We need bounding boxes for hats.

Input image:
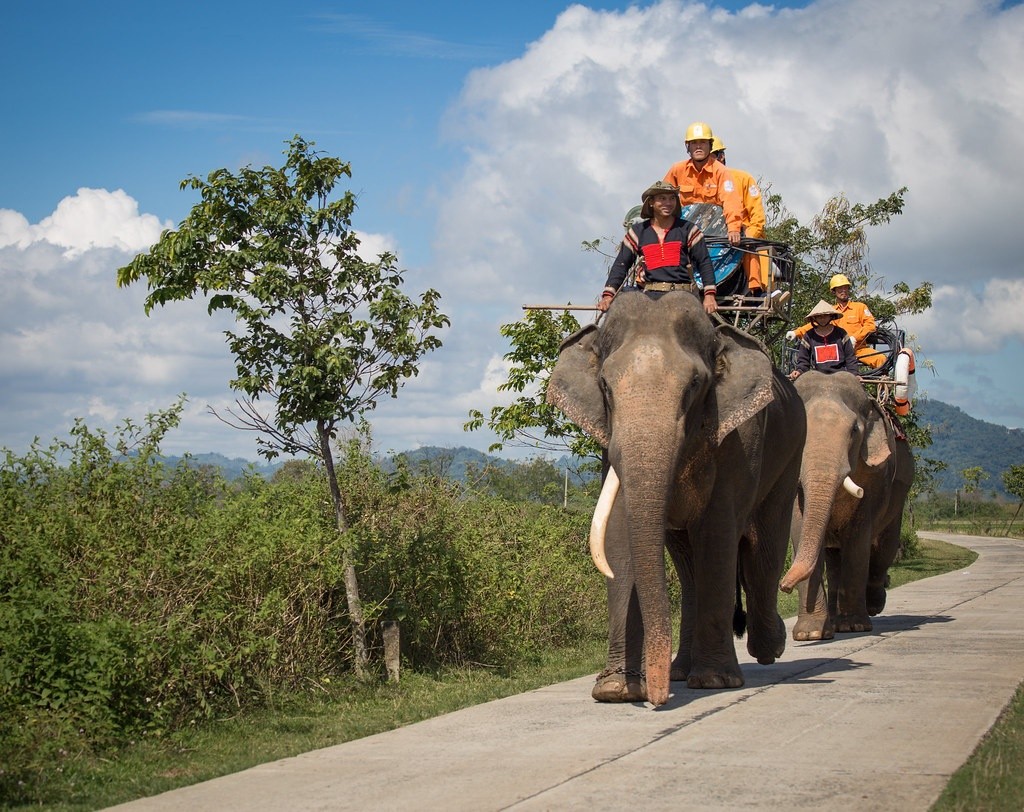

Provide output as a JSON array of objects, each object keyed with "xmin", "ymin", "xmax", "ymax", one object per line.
[
  {"xmin": 640, "ymin": 181, "xmax": 682, "ymax": 218},
  {"xmin": 805, "ymin": 300, "xmax": 843, "ymax": 321}
]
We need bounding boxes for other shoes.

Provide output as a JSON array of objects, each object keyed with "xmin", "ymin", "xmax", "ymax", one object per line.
[
  {"xmin": 881, "ymin": 388, "xmax": 891, "ymax": 400},
  {"xmin": 754, "ymin": 290, "xmax": 781, "ymax": 310},
  {"xmin": 776, "ymin": 291, "xmax": 791, "ymax": 306}
]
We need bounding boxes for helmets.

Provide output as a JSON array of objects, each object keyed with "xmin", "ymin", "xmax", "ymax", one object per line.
[
  {"xmin": 830, "ymin": 275, "xmax": 851, "ymax": 291},
  {"xmin": 684, "ymin": 122, "xmax": 714, "ymax": 142},
  {"xmin": 710, "ymin": 137, "xmax": 726, "ymax": 153}
]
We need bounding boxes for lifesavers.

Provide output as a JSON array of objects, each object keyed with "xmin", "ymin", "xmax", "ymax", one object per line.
[{"xmin": 894, "ymin": 348, "xmax": 916, "ymax": 415}]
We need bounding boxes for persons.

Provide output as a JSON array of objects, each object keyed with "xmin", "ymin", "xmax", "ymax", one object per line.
[
  {"xmin": 709, "ymin": 137, "xmax": 772, "ymax": 293},
  {"xmin": 788, "ymin": 300, "xmax": 863, "ymax": 384},
  {"xmin": 661, "ymin": 122, "xmax": 784, "ymax": 300},
  {"xmin": 785, "ymin": 274, "xmax": 892, "ymax": 397},
  {"xmin": 597, "ymin": 181, "xmax": 719, "ymax": 313}
]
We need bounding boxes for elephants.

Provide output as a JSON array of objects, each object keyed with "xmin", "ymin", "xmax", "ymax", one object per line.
[{"xmin": 544, "ymin": 290, "xmax": 916, "ymax": 706}]
